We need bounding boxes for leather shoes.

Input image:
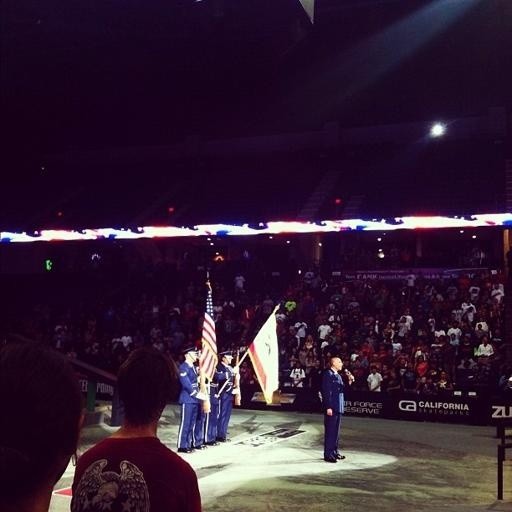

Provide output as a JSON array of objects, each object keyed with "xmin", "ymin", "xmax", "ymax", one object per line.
[
  {"xmin": 335, "ymin": 454, "xmax": 345, "ymax": 459},
  {"xmin": 324, "ymin": 455, "xmax": 337, "ymax": 463},
  {"xmin": 177, "ymin": 437, "xmax": 231, "ymax": 453}
]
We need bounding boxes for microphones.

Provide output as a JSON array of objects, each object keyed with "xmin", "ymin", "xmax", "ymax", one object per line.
[{"xmin": 345, "ymin": 369, "xmax": 355, "ymax": 382}]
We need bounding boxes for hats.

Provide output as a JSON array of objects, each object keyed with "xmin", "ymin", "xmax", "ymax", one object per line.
[
  {"xmin": 182, "ymin": 345, "xmax": 198, "ymax": 355},
  {"xmin": 219, "ymin": 350, "xmax": 233, "ymax": 357}
]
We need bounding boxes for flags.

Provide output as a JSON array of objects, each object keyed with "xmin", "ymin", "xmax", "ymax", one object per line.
[
  {"xmin": 198, "ymin": 282, "xmax": 218, "ymax": 381},
  {"xmin": 248, "ymin": 313, "xmax": 278, "ymax": 403}
]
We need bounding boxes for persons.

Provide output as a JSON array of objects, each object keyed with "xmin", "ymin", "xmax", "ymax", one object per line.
[
  {"xmin": 321, "ymin": 357, "xmax": 344, "ymax": 462},
  {"xmin": 1, "ymin": 330, "xmax": 239, "ymax": 511},
  {"xmin": 2, "ymin": 237, "xmax": 510, "ymax": 390}
]
